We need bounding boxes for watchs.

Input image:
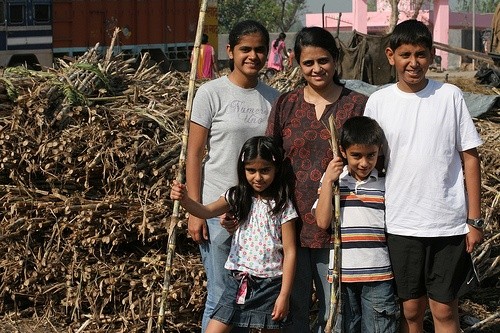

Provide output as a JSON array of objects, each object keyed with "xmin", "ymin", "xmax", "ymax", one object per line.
[{"xmin": 466, "ymin": 217, "xmax": 485, "ymax": 229}]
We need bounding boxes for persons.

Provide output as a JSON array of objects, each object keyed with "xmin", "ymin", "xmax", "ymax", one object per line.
[
  {"xmin": 170, "ymin": 136, "xmax": 300, "ymax": 333},
  {"xmin": 186, "ymin": 20, "xmax": 282, "ymax": 333},
  {"xmin": 221, "ymin": 27, "xmax": 369, "ymax": 333},
  {"xmin": 311, "ymin": 116, "xmax": 398, "ymax": 333},
  {"xmin": 190, "ymin": 32, "xmax": 294, "ymax": 80},
  {"xmin": 362, "ymin": 19, "xmax": 484, "ymax": 333}
]
